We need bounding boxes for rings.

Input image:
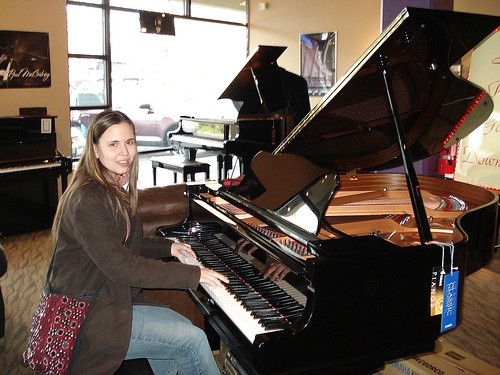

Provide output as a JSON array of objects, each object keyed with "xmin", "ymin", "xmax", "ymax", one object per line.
[
  {"xmin": 243, "ymin": 242, "xmax": 247, "ymax": 245},
  {"xmin": 185, "ymin": 247, "xmax": 189, "ymax": 252},
  {"xmin": 210, "ymin": 271, "xmax": 214, "ymax": 276}
]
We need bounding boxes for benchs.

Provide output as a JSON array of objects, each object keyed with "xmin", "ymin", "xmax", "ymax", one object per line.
[{"xmin": 150, "ymin": 157, "xmax": 211, "ymax": 186}]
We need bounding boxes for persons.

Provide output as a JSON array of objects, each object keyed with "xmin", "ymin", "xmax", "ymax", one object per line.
[
  {"xmin": 41, "ymin": 109, "xmax": 231, "ymax": 375},
  {"xmin": 233, "ymin": 238, "xmax": 291, "ymax": 282}
]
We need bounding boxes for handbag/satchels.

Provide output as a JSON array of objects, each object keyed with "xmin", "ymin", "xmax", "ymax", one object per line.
[{"xmin": 20, "ymin": 281, "xmax": 91, "ymax": 375}]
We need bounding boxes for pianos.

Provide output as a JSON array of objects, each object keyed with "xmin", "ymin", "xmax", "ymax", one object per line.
[
  {"xmin": 163, "ymin": 45, "xmax": 311, "ymax": 180},
  {"xmin": 0, "ymin": 114, "xmax": 73, "ymax": 236},
  {"xmin": 155, "ymin": 6, "xmax": 500, "ymax": 375}
]
